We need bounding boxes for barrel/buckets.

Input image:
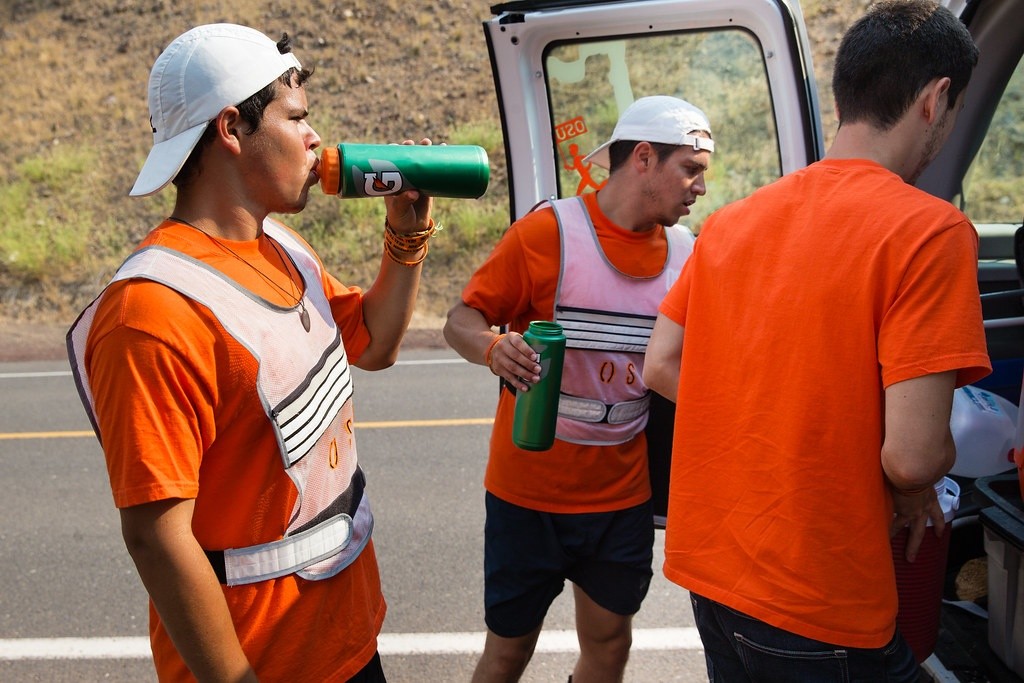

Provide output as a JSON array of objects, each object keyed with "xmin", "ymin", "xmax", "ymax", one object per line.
[{"xmin": 946, "ymin": 384, "xmax": 1019, "ymax": 478}]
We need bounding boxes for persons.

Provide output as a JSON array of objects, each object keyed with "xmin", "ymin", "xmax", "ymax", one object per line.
[
  {"xmin": 442, "ymin": 95, "xmax": 714, "ymax": 683},
  {"xmin": 85, "ymin": 24, "xmax": 447, "ymax": 682},
  {"xmin": 641, "ymin": 0, "xmax": 993, "ymax": 683}
]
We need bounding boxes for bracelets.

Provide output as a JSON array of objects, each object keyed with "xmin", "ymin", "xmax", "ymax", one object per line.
[
  {"xmin": 484, "ymin": 334, "xmax": 506, "ymax": 367},
  {"xmin": 383, "ymin": 216, "xmax": 435, "ymax": 269}
]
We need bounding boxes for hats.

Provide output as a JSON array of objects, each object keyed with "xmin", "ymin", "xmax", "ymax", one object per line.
[
  {"xmin": 582, "ymin": 96, "xmax": 714, "ymax": 170},
  {"xmin": 129, "ymin": 23, "xmax": 303, "ymax": 197}
]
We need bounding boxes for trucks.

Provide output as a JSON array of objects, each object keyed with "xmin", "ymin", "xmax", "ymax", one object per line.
[{"xmin": 483, "ymin": 1, "xmax": 1024, "ymax": 683}]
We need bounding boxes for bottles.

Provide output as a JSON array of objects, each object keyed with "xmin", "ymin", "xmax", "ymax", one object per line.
[
  {"xmin": 314, "ymin": 143, "xmax": 490, "ymax": 198},
  {"xmin": 512, "ymin": 321, "xmax": 566, "ymax": 451}
]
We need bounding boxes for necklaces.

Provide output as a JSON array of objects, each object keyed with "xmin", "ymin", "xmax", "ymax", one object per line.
[{"xmin": 171, "ymin": 215, "xmax": 311, "ymax": 333}]
